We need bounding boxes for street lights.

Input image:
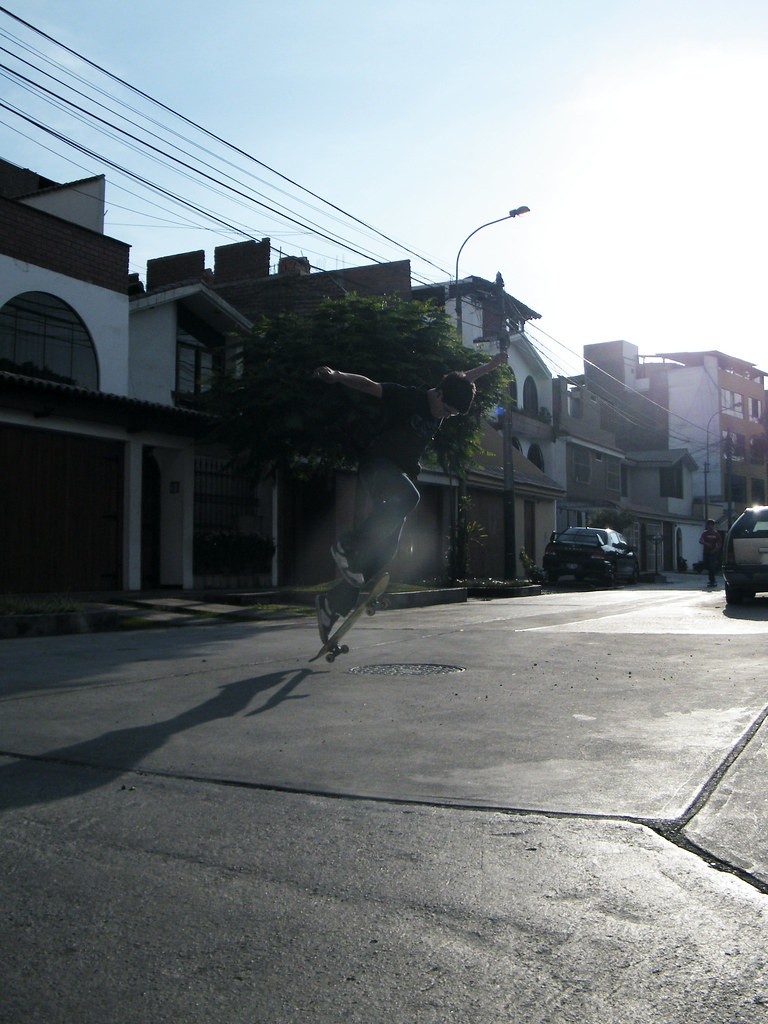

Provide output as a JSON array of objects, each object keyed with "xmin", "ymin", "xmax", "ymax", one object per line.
[
  {"xmin": 703, "ymin": 401, "xmax": 743, "ymax": 531},
  {"xmin": 446, "ymin": 206, "xmax": 529, "ymax": 582}
]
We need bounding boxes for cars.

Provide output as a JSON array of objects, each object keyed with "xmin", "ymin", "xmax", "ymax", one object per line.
[{"xmin": 542, "ymin": 525, "xmax": 641, "ymax": 587}]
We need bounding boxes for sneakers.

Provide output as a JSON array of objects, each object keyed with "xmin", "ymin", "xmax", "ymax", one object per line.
[
  {"xmin": 331, "ymin": 543, "xmax": 366, "ymax": 588},
  {"xmin": 315, "ymin": 593, "xmax": 340, "ymax": 644}
]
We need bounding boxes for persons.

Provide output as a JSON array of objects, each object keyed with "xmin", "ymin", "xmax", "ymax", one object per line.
[
  {"xmin": 699, "ymin": 519, "xmax": 723, "ymax": 584},
  {"xmin": 312, "ymin": 352, "xmax": 509, "ymax": 646}
]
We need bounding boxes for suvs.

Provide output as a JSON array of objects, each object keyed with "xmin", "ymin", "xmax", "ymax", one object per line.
[{"xmin": 721, "ymin": 506, "xmax": 768, "ymax": 605}]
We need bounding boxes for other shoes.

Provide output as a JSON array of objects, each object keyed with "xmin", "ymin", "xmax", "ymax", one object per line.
[{"xmin": 708, "ymin": 582, "xmax": 717, "ymax": 587}]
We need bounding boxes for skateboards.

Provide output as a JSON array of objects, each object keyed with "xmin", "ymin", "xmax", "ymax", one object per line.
[
  {"xmin": 707, "ymin": 581, "xmax": 717, "ymax": 587},
  {"xmin": 307, "ymin": 572, "xmax": 390, "ymax": 663}
]
